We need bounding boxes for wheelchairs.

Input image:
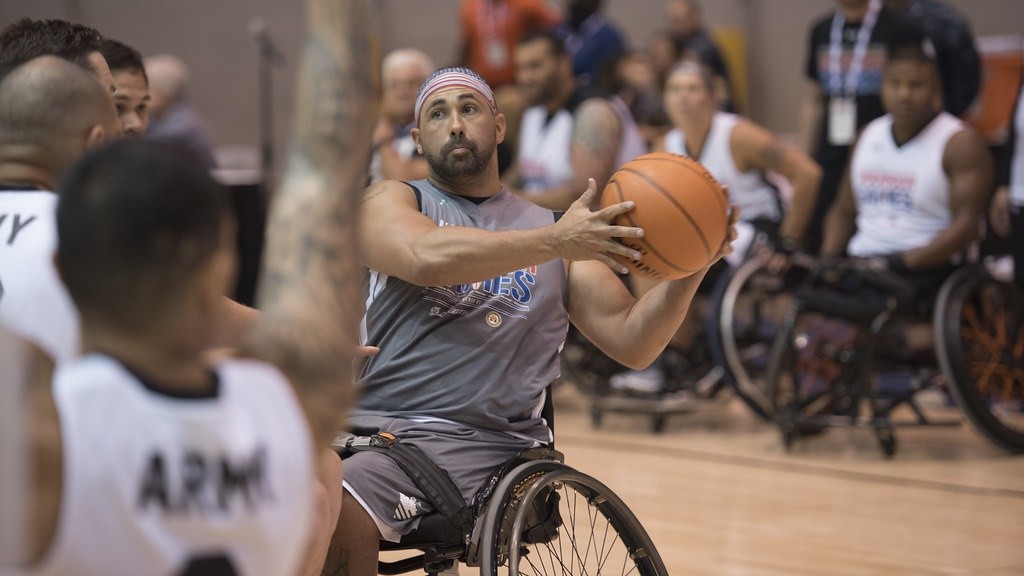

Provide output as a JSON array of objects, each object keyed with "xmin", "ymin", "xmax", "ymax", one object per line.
[
  {"xmin": 369, "ymin": 383, "xmax": 669, "ymax": 576},
  {"xmin": 589, "ymin": 217, "xmax": 1024, "ymax": 457}
]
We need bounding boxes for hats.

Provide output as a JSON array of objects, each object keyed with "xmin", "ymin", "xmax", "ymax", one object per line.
[{"xmin": 415, "ymin": 68, "xmax": 498, "ymax": 130}]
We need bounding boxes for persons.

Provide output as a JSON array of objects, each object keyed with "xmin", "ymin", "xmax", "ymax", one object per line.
[{"xmin": 0, "ymin": 0, "xmax": 1024, "ymax": 576}]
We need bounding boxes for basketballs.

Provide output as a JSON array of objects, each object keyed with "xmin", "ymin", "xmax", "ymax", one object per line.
[{"xmin": 598, "ymin": 150, "xmax": 729, "ymax": 283}]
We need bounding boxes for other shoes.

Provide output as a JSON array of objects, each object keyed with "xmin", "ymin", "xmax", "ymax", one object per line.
[{"xmin": 609, "ymin": 368, "xmax": 666, "ymax": 396}]
